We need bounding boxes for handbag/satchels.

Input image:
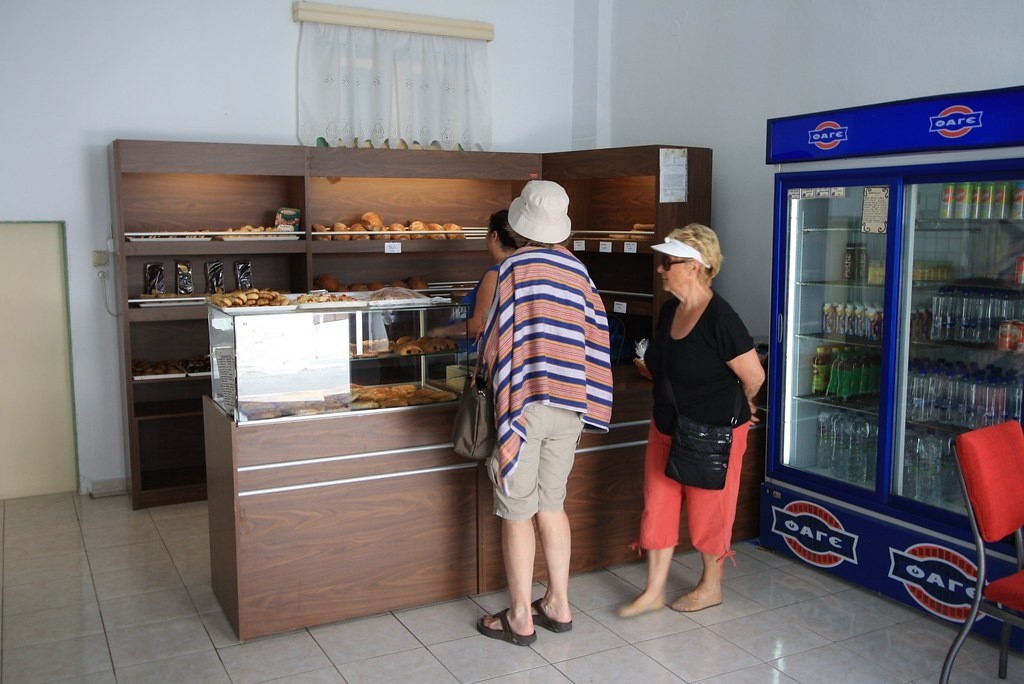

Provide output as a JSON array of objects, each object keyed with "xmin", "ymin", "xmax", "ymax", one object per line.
[
  {"xmin": 453, "ymin": 376, "xmax": 496, "ymax": 461},
  {"xmin": 664, "ymin": 414, "xmax": 734, "ymax": 491}
]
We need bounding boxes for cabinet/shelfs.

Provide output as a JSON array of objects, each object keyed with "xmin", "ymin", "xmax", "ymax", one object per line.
[{"xmin": 108, "ymin": 139, "xmax": 711, "ymax": 513}]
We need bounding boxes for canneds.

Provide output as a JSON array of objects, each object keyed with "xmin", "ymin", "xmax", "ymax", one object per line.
[
  {"xmin": 1013, "ymin": 256, "xmax": 1024, "ymax": 287},
  {"xmin": 939, "ymin": 181, "xmax": 1024, "ymax": 220},
  {"xmin": 997, "ymin": 319, "xmax": 1024, "ymax": 352}
]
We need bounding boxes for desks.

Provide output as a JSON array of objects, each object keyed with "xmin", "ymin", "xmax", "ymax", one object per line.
[
  {"xmin": 426, "ymin": 374, "xmax": 762, "ymax": 595},
  {"xmin": 201, "ymin": 381, "xmax": 479, "ymax": 642}
]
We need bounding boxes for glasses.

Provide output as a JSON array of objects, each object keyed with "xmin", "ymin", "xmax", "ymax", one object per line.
[{"xmin": 660, "ymin": 253, "xmax": 686, "ymax": 271}]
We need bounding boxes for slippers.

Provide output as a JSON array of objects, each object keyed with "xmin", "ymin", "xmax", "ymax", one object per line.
[
  {"xmin": 531, "ymin": 598, "xmax": 573, "ymax": 633},
  {"xmin": 476, "ymin": 608, "xmax": 537, "ymax": 646}
]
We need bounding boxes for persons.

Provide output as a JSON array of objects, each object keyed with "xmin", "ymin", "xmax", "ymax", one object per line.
[
  {"xmin": 615, "ymin": 225, "xmax": 765, "ymax": 617},
  {"xmin": 424, "ymin": 209, "xmax": 520, "ymax": 381},
  {"xmin": 476, "ymin": 179, "xmax": 612, "ymax": 646}
]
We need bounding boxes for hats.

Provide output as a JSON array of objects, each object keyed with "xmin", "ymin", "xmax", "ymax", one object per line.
[
  {"xmin": 508, "ymin": 180, "xmax": 572, "ymax": 243},
  {"xmin": 650, "ymin": 237, "xmax": 711, "ymax": 269}
]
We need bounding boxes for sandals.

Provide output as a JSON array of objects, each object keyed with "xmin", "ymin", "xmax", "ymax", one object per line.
[
  {"xmin": 617, "ymin": 595, "xmax": 665, "ymax": 618},
  {"xmin": 671, "ymin": 590, "xmax": 723, "ymax": 612}
]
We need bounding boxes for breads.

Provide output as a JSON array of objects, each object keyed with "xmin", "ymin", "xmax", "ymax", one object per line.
[
  {"xmin": 132, "ymin": 212, "xmax": 477, "ymax": 420},
  {"xmin": 609, "ymin": 223, "xmax": 655, "ymax": 240}
]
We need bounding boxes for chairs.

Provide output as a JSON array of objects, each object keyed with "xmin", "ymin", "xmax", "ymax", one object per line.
[{"xmin": 938, "ymin": 420, "xmax": 1024, "ymax": 683}]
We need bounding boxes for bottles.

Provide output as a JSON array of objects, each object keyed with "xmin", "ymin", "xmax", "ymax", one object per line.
[
  {"xmin": 844, "ymin": 243, "xmax": 868, "ymax": 284},
  {"xmin": 823, "ymin": 302, "xmax": 931, "ymax": 343},
  {"xmin": 906, "ymin": 357, "xmax": 1024, "ymax": 429},
  {"xmin": 812, "ymin": 347, "xmax": 881, "ymax": 403},
  {"xmin": 930, "ymin": 286, "xmax": 1024, "ymax": 345},
  {"xmin": 904, "ymin": 423, "xmax": 964, "ymax": 506},
  {"xmin": 816, "ymin": 406, "xmax": 879, "ymax": 483}
]
